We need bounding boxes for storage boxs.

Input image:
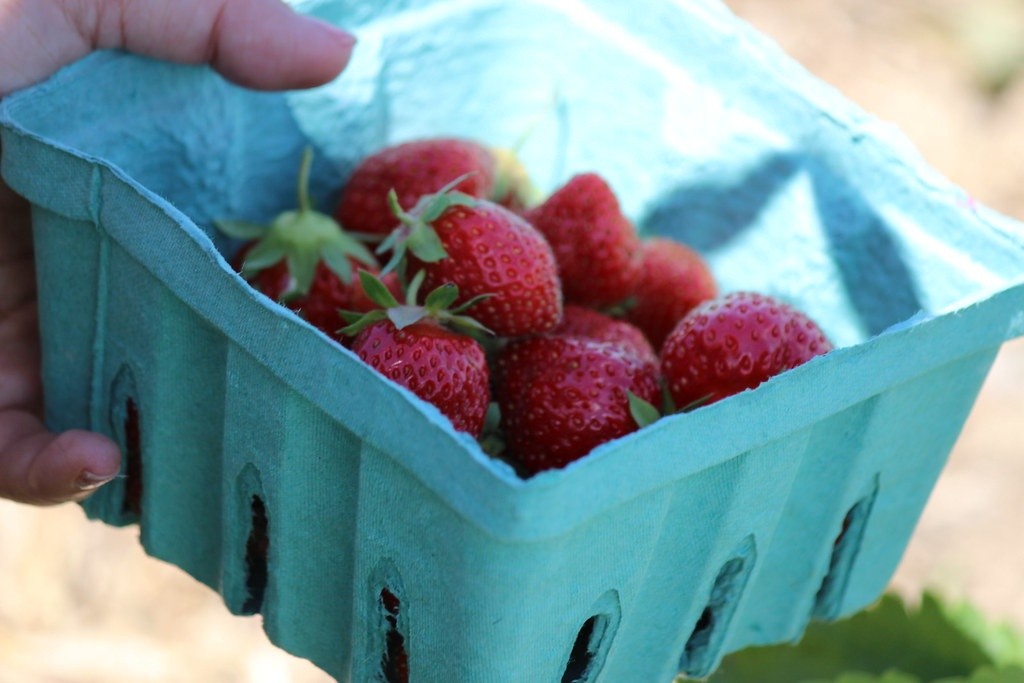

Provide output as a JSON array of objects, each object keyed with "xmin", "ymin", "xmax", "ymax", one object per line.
[{"xmin": 0, "ymin": 0, "xmax": 1024, "ymax": 683}]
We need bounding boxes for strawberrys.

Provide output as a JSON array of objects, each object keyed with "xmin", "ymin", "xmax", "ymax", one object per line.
[{"xmin": 210, "ymin": 139, "xmax": 840, "ymax": 481}]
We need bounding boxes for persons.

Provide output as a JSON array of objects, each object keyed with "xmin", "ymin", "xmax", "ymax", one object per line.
[{"xmin": 0, "ymin": 0, "xmax": 360, "ymax": 509}]
